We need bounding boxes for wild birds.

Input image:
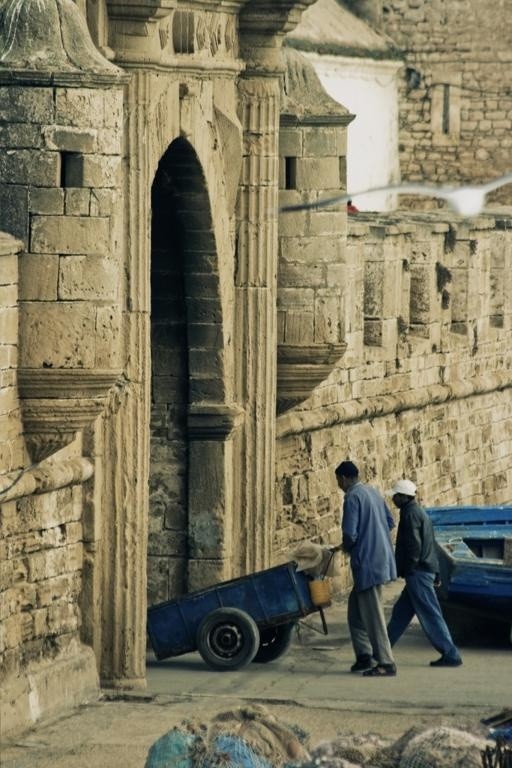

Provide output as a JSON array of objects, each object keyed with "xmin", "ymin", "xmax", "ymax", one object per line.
[{"xmin": 277, "ymin": 173, "xmax": 512, "ymax": 222}]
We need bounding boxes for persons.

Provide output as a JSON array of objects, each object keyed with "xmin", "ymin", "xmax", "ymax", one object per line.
[
  {"xmin": 334, "ymin": 457, "xmax": 402, "ymax": 678},
  {"xmin": 369, "ymin": 477, "xmax": 463, "ymax": 667}
]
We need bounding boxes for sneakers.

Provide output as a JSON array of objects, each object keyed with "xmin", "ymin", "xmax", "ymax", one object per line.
[
  {"xmin": 350, "ymin": 657, "xmax": 373, "ymax": 672},
  {"xmin": 363, "ymin": 666, "xmax": 397, "ymax": 677},
  {"xmin": 430, "ymin": 655, "xmax": 462, "ymax": 667}
]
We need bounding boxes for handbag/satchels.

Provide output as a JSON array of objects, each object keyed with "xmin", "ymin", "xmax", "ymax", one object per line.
[{"xmin": 308, "ymin": 580, "xmax": 334, "ymax": 606}]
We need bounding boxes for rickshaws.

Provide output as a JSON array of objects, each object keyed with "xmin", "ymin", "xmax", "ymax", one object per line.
[{"xmin": 147, "ymin": 544, "xmax": 343, "ymax": 671}]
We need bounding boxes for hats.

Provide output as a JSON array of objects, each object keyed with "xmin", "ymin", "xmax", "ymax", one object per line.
[{"xmin": 384, "ymin": 479, "xmax": 417, "ymax": 498}]
